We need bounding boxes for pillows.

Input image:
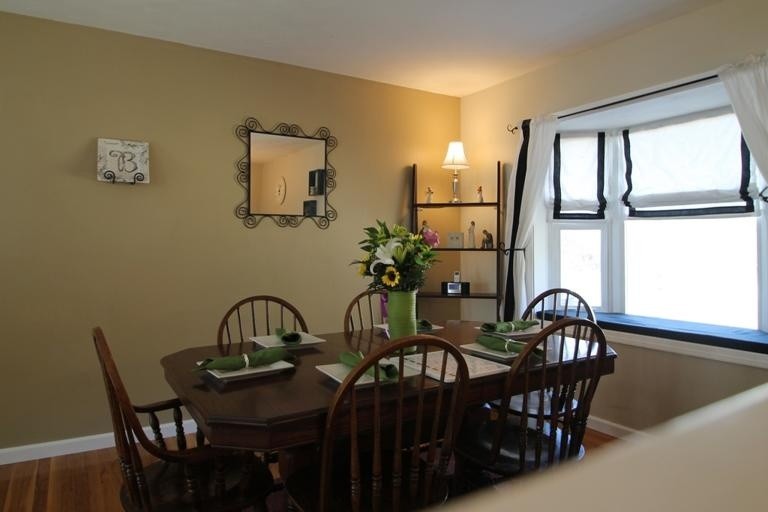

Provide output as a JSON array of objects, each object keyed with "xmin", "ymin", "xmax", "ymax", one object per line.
[
  {"xmin": 192, "ymin": 347, "xmax": 288, "ymax": 372},
  {"xmin": 417, "ymin": 318, "xmax": 432, "ymax": 331},
  {"xmin": 477, "ymin": 336, "xmax": 547, "ymax": 362},
  {"xmin": 277, "ymin": 328, "xmax": 302, "ymax": 344},
  {"xmin": 481, "ymin": 321, "xmax": 539, "ymax": 333},
  {"xmin": 340, "ymin": 349, "xmax": 398, "ymax": 379}
]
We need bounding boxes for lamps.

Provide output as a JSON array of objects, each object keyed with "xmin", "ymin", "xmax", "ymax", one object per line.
[{"xmin": 442, "ymin": 142, "xmax": 469, "ymax": 202}]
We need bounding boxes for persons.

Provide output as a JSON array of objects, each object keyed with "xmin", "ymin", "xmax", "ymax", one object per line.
[
  {"xmin": 468, "ymin": 221, "xmax": 476, "ymax": 249},
  {"xmin": 420, "ymin": 220, "xmax": 439, "ymax": 248},
  {"xmin": 479, "ymin": 230, "xmax": 493, "ymax": 249},
  {"xmin": 425, "ymin": 186, "xmax": 434, "ymax": 202},
  {"xmin": 476, "ymin": 186, "xmax": 483, "ymax": 203}
]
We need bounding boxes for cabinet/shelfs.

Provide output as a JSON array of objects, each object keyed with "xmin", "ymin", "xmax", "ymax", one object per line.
[{"xmin": 410, "ymin": 161, "xmax": 504, "ymax": 321}]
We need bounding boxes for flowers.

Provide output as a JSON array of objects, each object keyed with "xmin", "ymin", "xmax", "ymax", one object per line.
[{"xmin": 350, "ymin": 218, "xmax": 437, "ymax": 291}]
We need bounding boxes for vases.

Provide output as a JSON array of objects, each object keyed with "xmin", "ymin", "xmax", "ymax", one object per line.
[{"xmin": 387, "ymin": 292, "xmax": 418, "ymax": 352}]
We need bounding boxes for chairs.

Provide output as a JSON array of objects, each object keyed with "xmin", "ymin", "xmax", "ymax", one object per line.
[
  {"xmin": 489, "ymin": 289, "xmax": 592, "ymax": 418},
  {"xmin": 458, "ymin": 317, "xmax": 606, "ymax": 494},
  {"xmin": 283, "ymin": 334, "xmax": 469, "ymax": 512},
  {"xmin": 92, "ymin": 327, "xmax": 274, "ymax": 512},
  {"xmin": 345, "ymin": 291, "xmax": 380, "ymax": 331},
  {"xmin": 217, "ymin": 296, "xmax": 308, "ymax": 491}
]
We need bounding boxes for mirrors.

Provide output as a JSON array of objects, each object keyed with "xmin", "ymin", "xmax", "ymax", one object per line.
[{"xmin": 234, "ymin": 117, "xmax": 337, "ymax": 228}]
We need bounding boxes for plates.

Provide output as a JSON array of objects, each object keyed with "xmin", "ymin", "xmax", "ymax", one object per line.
[
  {"xmin": 315, "ymin": 357, "xmax": 423, "ymax": 389},
  {"xmin": 472, "ymin": 325, "xmax": 545, "ymax": 339},
  {"xmin": 248, "ymin": 331, "xmax": 328, "ymax": 352},
  {"xmin": 195, "ymin": 353, "xmax": 298, "ymax": 384},
  {"xmin": 373, "ymin": 321, "xmax": 446, "ymax": 335},
  {"xmin": 459, "ymin": 341, "xmax": 553, "ymax": 363}
]
